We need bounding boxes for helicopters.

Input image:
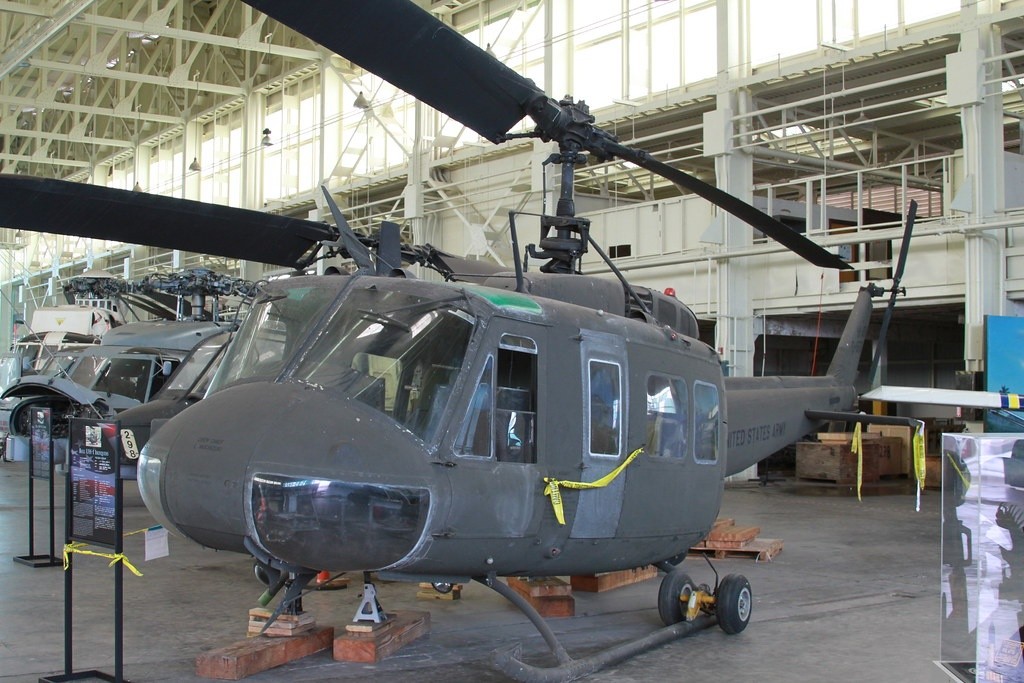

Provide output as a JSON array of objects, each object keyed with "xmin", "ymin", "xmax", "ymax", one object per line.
[{"xmin": 0, "ymin": 0, "xmax": 1024, "ymax": 683}]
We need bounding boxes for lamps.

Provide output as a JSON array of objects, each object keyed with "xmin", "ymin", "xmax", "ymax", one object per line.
[
  {"xmin": 354, "ymin": 68, "xmax": 368, "ymax": 108},
  {"xmin": 189, "ymin": 75, "xmax": 201, "ymax": 171},
  {"xmin": 132, "ymin": 112, "xmax": 142, "ymax": 191},
  {"xmin": 261, "ymin": 36, "xmax": 273, "ymax": 145}
]
